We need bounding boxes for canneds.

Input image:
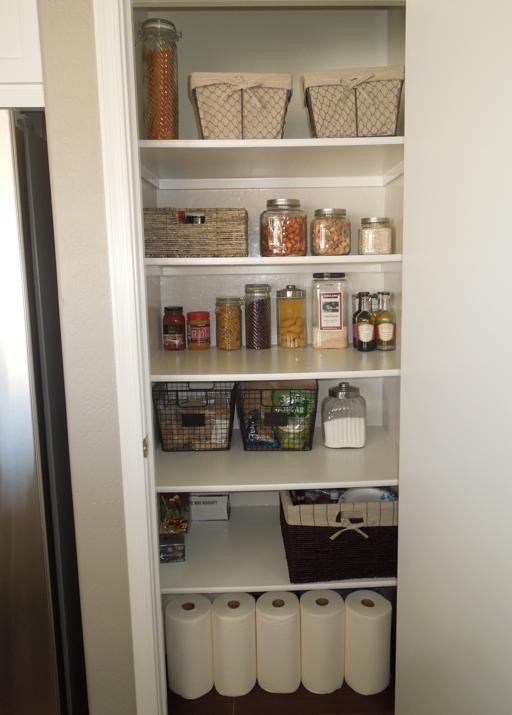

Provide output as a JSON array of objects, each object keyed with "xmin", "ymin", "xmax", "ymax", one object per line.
[{"xmin": 187, "ymin": 312, "xmax": 211, "ymax": 347}]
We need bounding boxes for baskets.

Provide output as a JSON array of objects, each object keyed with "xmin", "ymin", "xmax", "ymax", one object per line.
[
  {"xmin": 152, "ymin": 382, "xmax": 235, "ymax": 452},
  {"xmin": 188, "ymin": 72, "xmax": 293, "ymax": 138},
  {"xmin": 236, "ymin": 380, "xmax": 318, "ymax": 451},
  {"xmin": 301, "ymin": 65, "xmax": 404, "ymax": 138}
]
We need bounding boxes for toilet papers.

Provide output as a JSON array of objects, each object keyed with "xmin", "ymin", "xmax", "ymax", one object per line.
[
  {"xmin": 211, "ymin": 593, "xmax": 257, "ymax": 698},
  {"xmin": 163, "ymin": 594, "xmax": 214, "ymax": 701},
  {"xmin": 345, "ymin": 589, "xmax": 393, "ymax": 696},
  {"xmin": 255, "ymin": 591, "xmax": 302, "ymax": 696},
  {"xmin": 299, "ymin": 590, "xmax": 345, "ymax": 695}
]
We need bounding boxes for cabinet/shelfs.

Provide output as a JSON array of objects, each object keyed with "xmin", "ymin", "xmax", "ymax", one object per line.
[{"xmin": 91, "ymin": 2, "xmax": 406, "ymax": 715}]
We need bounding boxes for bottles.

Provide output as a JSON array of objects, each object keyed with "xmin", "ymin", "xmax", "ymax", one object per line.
[
  {"xmin": 215, "ymin": 296, "xmax": 242, "ymax": 351},
  {"xmin": 244, "ymin": 284, "xmax": 272, "ymax": 351},
  {"xmin": 321, "ymin": 382, "xmax": 366, "ymax": 451},
  {"xmin": 352, "ymin": 288, "xmax": 397, "ymax": 353},
  {"xmin": 276, "ymin": 284, "xmax": 308, "ymax": 351},
  {"xmin": 134, "ymin": 20, "xmax": 182, "ymax": 140},
  {"xmin": 259, "ymin": 198, "xmax": 308, "ymax": 257},
  {"xmin": 310, "ymin": 206, "xmax": 352, "ymax": 255},
  {"xmin": 357, "ymin": 215, "xmax": 394, "ymax": 255},
  {"xmin": 185, "ymin": 310, "xmax": 212, "ymax": 350},
  {"xmin": 311, "ymin": 271, "xmax": 351, "ymax": 351}
]
